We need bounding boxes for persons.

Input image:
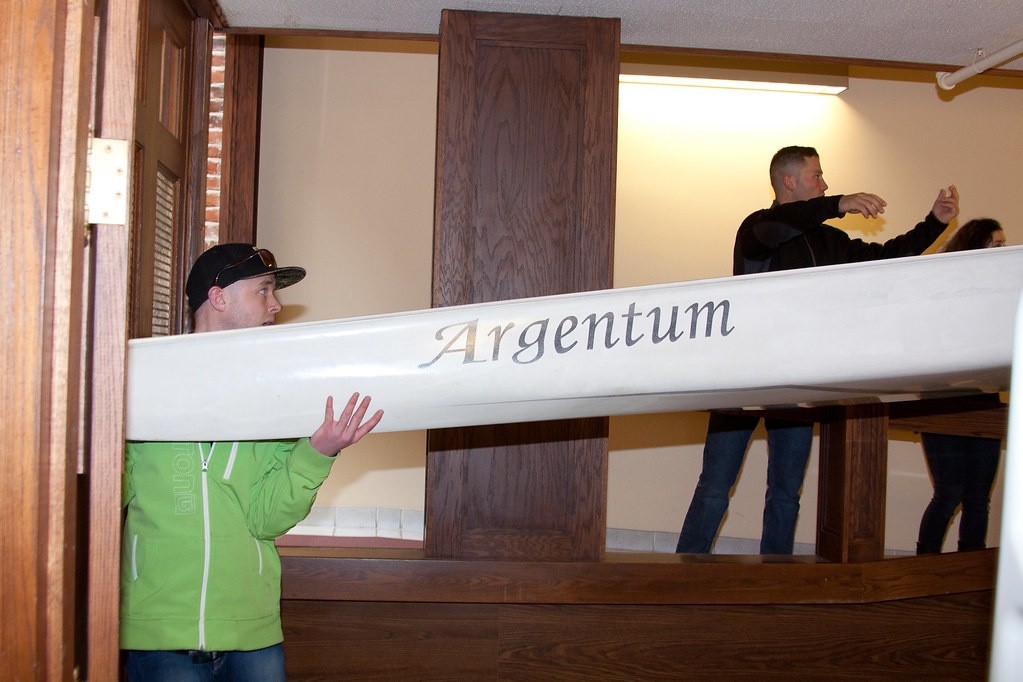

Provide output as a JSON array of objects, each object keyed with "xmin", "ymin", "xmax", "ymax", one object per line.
[
  {"xmin": 116, "ymin": 242, "xmax": 383, "ymax": 682},
  {"xmin": 676, "ymin": 145, "xmax": 959, "ymax": 555},
  {"xmin": 918, "ymin": 219, "xmax": 1006, "ymax": 556}
]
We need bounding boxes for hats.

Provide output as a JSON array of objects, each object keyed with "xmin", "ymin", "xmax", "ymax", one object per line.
[{"xmin": 186, "ymin": 243, "xmax": 306, "ymax": 313}]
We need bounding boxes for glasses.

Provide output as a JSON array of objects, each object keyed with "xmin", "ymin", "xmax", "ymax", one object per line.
[{"xmin": 215, "ymin": 249, "xmax": 277, "ymax": 285}]
